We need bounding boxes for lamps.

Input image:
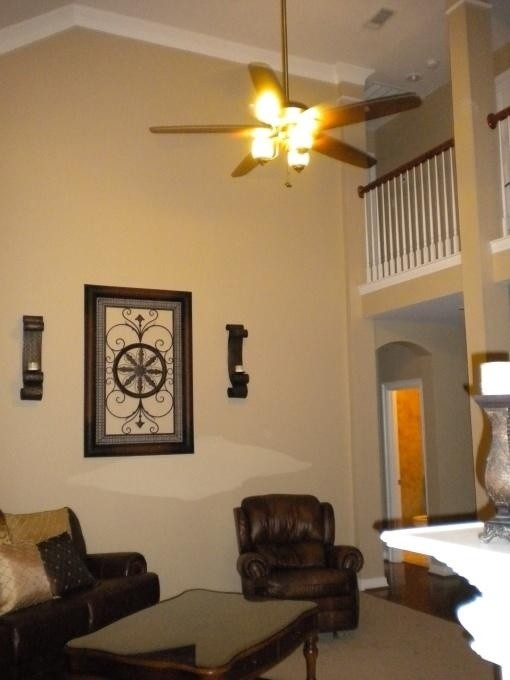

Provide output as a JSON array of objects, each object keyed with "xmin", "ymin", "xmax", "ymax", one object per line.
[
  {"xmin": 251, "ymin": 0, "xmax": 322, "ymax": 174},
  {"xmin": 20, "ymin": 315, "xmax": 249, "ymax": 400}
]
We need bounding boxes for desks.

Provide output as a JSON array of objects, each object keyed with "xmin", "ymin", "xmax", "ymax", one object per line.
[{"xmin": 380, "ymin": 521, "xmax": 510, "ymax": 680}]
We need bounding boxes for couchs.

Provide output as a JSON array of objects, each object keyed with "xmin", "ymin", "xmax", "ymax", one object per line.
[{"xmin": 0, "ymin": 507, "xmax": 160, "ymax": 680}]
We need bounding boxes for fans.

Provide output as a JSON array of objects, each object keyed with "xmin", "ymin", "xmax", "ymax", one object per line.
[{"xmin": 151, "ymin": 0, "xmax": 422, "ymax": 178}]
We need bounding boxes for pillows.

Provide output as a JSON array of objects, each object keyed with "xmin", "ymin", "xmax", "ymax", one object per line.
[{"xmin": 0, "ymin": 533, "xmax": 99, "ymax": 616}]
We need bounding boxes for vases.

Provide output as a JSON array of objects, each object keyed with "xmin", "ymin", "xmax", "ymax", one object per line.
[{"xmin": 475, "ymin": 395, "xmax": 510, "ymax": 545}]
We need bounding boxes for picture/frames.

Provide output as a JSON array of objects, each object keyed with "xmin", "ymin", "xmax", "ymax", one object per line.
[{"xmin": 84, "ymin": 282, "xmax": 193, "ymax": 457}]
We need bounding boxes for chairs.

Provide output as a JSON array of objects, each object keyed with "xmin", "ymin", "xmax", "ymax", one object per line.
[{"xmin": 233, "ymin": 495, "xmax": 365, "ymax": 635}]
